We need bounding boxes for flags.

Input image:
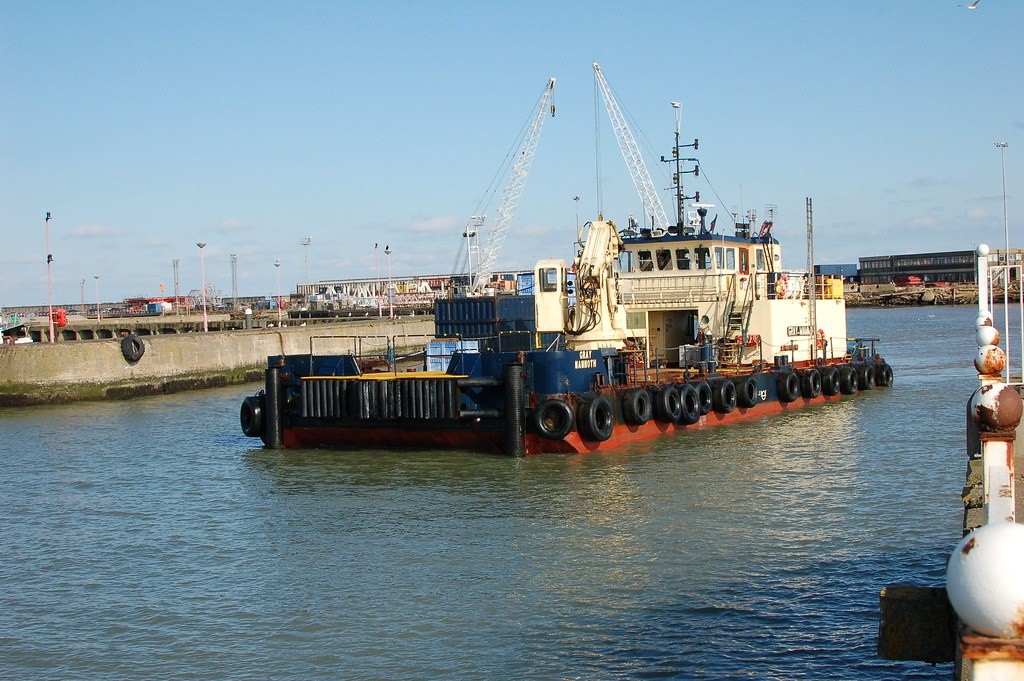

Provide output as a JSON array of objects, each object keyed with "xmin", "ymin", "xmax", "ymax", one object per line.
[{"xmin": 709, "ymin": 217, "xmax": 716, "ymax": 232}]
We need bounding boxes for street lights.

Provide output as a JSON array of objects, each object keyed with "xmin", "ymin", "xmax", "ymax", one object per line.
[
  {"xmin": 94, "ymin": 276, "xmax": 100, "ymax": 322},
  {"xmin": 45, "ymin": 212, "xmax": 54, "ymax": 343},
  {"xmin": 274, "ymin": 263, "xmax": 281, "ymax": 328},
  {"xmin": 574, "ymin": 197, "xmax": 580, "ymax": 230},
  {"xmin": 384, "ymin": 245, "xmax": 393, "ymax": 318},
  {"xmin": 375, "ymin": 243, "xmax": 382, "ymax": 317},
  {"xmin": 197, "ymin": 242, "xmax": 207, "ymax": 332},
  {"xmin": 996, "ymin": 142, "xmax": 1010, "ymax": 284}
]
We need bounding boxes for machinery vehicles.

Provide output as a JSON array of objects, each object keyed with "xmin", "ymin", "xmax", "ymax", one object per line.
[
  {"xmin": 451, "ymin": 77, "xmax": 556, "ymax": 298},
  {"xmin": 592, "ymin": 62, "xmax": 699, "ymax": 272}
]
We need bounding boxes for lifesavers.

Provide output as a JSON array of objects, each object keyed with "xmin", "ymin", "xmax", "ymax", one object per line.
[
  {"xmin": 577, "ymin": 395, "xmax": 614, "ymax": 443},
  {"xmin": 655, "ymin": 375, "xmax": 761, "ymax": 426},
  {"xmin": 120, "ymin": 334, "xmax": 147, "ymax": 362},
  {"xmin": 531, "ymin": 399, "xmax": 574, "ymax": 440},
  {"xmin": 816, "ymin": 328, "xmax": 825, "ymax": 349},
  {"xmin": 239, "ymin": 395, "xmax": 261, "ymax": 438},
  {"xmin": 775, "ymin": 362, "xmax": 894, "ymax": 404},
  {"xmin": 621, "ymin": 388, "xmax": 652, "ymax": 427}
]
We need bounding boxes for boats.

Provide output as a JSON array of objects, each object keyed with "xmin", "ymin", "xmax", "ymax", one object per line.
[{"xmin": 240, "ymin": 101, "xmax": 893, "ymax": 458}]
